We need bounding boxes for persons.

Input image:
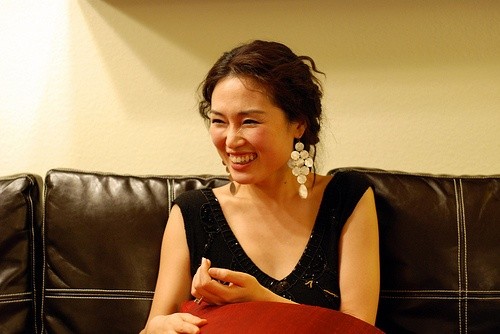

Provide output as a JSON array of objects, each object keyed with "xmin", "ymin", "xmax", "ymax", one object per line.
[{"xmin": 137, "ymin": 40, "xmax": 380, "ymax": 334}]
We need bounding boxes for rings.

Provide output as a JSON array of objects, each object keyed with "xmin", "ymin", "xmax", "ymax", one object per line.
[{"xmin": 194, "ymin": 296, "xmax": 205, "ymax": 305}]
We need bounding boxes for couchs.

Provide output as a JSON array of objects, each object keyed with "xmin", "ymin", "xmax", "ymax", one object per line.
[{"xmin": 0, "ymin": 167, "xmax": 500, "ymax": 334}]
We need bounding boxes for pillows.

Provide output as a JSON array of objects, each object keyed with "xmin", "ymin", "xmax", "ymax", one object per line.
[{"xmin": 177, "ymin": 299, "xmax": 386, "ymax": 334}]
